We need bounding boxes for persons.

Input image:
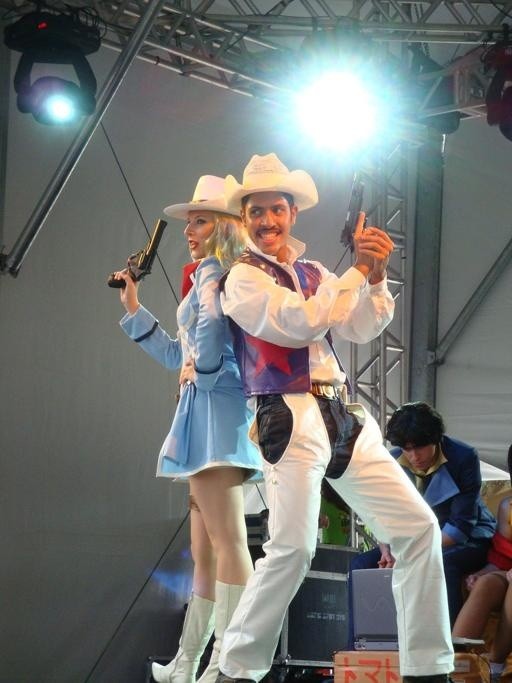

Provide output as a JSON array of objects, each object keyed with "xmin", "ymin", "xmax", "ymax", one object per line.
[
  {"xmin": 449, "ymin": 439, "xmax": 511, "ymax": 682},
  {"xmin": 362, "ymin": 400, "xmax": 496, "ymax": 627},
  {"xmin": 213, "ymin": 151, "xmax": 456, "ymax": 683},
  {"xmin": 111, "ymin": 174, "xmax": 263, "ymax": 683}
]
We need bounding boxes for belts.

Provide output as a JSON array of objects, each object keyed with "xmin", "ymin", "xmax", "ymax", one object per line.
[{"xmin": 310, "ymin": 382, "xmax": 346, "ymax": 405}]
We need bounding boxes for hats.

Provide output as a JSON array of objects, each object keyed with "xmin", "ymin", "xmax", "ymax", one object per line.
[
  {"xmin": 163, "ymin": 175, "xmax": 242, "ymax": 220},
  {"xmin": 223, "ymin": 152, "xmax": 319, "ymax": 216}
]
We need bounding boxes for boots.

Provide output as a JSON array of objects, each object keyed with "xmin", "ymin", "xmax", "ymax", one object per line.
[
  {"xmin": 196, "ymin": 579, "xmax": 246, "ymax": 683},
  {"xmin": 151, "ymin": 591, "xmax": 216, "ymax": 683}
]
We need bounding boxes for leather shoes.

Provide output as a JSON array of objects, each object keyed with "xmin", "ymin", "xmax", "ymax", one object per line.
[
  {"xmin": 215, "ymin": 671, "xmax": 256, "ymax": 683},
  {"xmin": 402, "ymin": 674, "xmax": 454, "ymax": 683}
]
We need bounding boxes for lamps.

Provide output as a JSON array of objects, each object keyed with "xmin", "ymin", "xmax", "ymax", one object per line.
[{"xmin": 4, "ymin": 10, "xmax": 100, "ymax": 128}]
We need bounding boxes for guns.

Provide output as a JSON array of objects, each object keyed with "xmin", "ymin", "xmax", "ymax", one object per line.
[
  {"xmin": 338, "ymin": 175, "xmax": 366, "ymax": 248},
  {"xmin": 108, "ymin": 220, "xmax": 166, "ymax": 289}
]
